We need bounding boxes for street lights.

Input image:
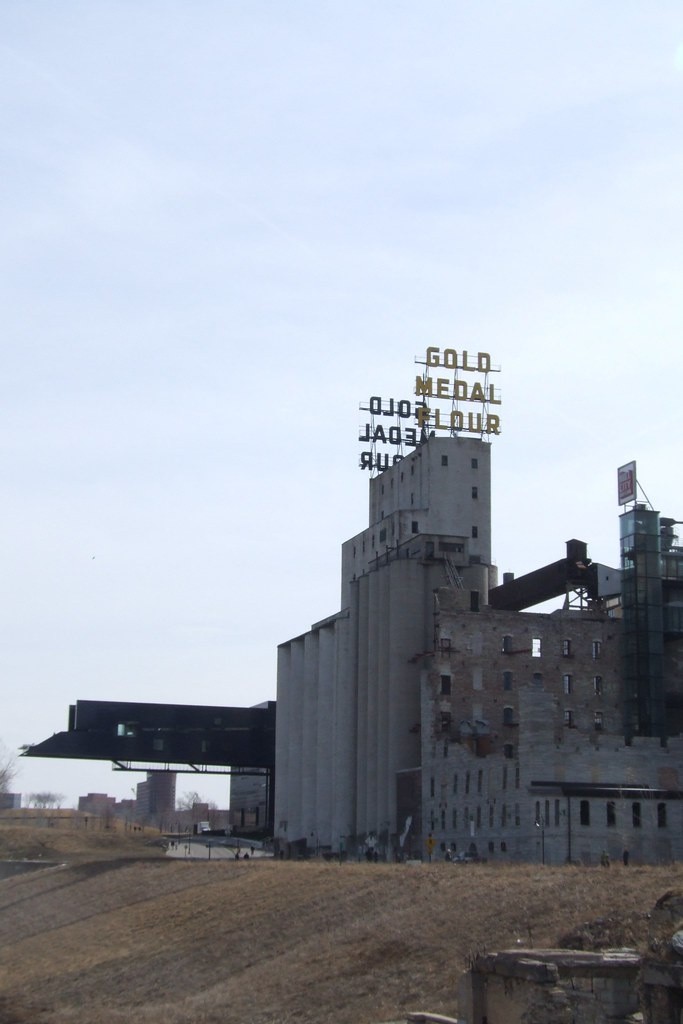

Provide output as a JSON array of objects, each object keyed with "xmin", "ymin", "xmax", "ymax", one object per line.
[
  {"xmin": 311, "ymin": 828, "xmax": 319, "ymax": 856},
  {"xmin": 535, "ymin": 817, "xmax": 546, "ymax": 866}
]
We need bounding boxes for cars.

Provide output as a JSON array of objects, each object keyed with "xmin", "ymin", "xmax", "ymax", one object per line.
[{"xmin": 452, "ymin": 851, "xmax": 486, "ymax": 864}]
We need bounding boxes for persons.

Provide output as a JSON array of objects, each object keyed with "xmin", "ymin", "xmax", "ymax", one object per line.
[
  {"xmin": 234, "ymin": 845, "xmax": 256, "ymax": 860},
  {"xmin": 623, "ymin": 846, "xmax": 629, "ymax": 866}
]
